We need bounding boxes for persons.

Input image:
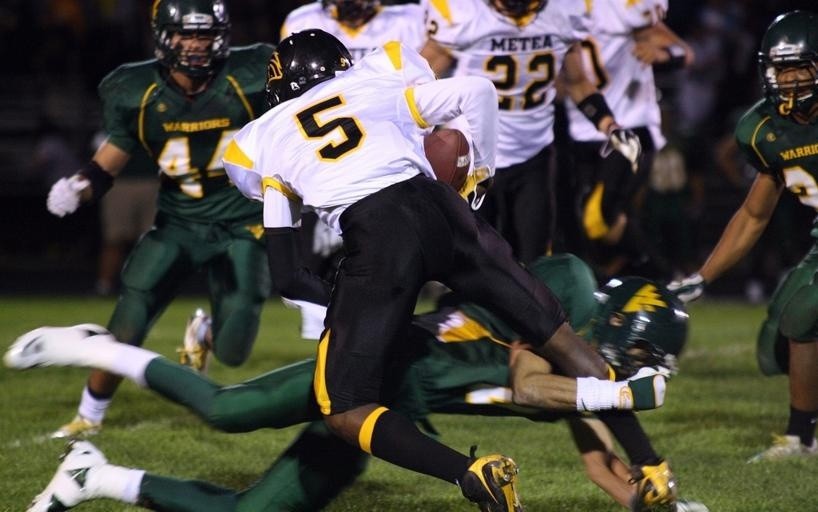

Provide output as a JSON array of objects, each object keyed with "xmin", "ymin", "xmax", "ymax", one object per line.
[
  {"xmin": 0, "ymin": 252, "xmax": 692, "ymax": 508},
  {"xmin": 219, "ymin": 25, "xmax": 682, "ymax": 508},
  {"xmin": 1, "ymin": 0, "xmax": 816, "ymax": 470}
]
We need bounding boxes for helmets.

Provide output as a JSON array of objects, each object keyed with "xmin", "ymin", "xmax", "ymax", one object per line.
[
  {"xmin": 151, "ymin": 0, "xmax": 231, "ymax": 78},
  {"xmin": 266, "ymin": 29, "xmax": 353, "ymax": 109},
  {"xmin": 595, "ymin": 278, "xmax": 687, "ymax": 371},
  {"xmin": 759, "ymin": 10, "xmax": 818, "ymax": 117}
]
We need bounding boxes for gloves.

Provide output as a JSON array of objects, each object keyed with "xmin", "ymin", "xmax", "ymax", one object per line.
[
  {"xmin": 600, "ymin": 127, "xmax": 641, "ymax": 162},
  {"xmin": 628, "ymin": 366, "xmax": 665, "ymax": 410}
]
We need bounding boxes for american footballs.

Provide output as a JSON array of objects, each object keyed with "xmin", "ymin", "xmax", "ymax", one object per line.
[{"xmin": 423, "ymin": 127, "xmax": 471, "ymax": 192}]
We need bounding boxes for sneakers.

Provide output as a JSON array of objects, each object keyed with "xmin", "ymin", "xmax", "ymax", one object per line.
[
  {"xmin": 179, "ymin": 309, "xmax": 211, "ymax": 371},
  {"xmin": 4, "ymin": 323, "xmax": 110, "ymax": 371},
  {"xmin": 27, "ymin": 414, "xmax": 106, "ymax": 512},
  {"xmin": 628, "ymin": 460, "xmax": 677, "ymax": 512},
  {"xmin": 47, "ymin": 176, "xmax": 81, "ymax": 217},
  {"xmin": 747, "ymin": 435, "xmax": 817, "ymax": 464},
  {"xmin": 458, "ymin": 445, "xmax": 523, "ymax": 512}
]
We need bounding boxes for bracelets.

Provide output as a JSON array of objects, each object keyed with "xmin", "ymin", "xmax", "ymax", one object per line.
[{"xmin": 572, "ymin": 374, "xmax": 622, "ymax": 421}]
